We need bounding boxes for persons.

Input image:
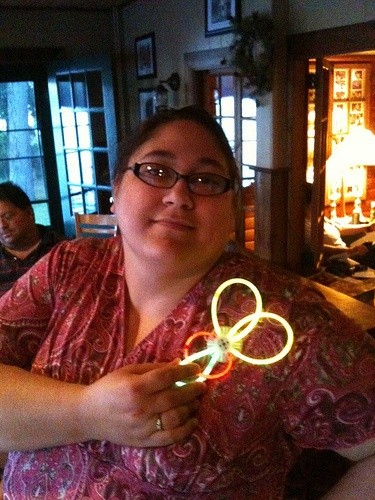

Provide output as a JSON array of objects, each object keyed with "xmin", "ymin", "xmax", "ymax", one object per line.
[
  {"xmin": 0, "ymin": 107, "xmax": 374, "ymax": 500},
  {"xmin": 0, "ymin": 181, "xmax": 67, "ymax": 299}
]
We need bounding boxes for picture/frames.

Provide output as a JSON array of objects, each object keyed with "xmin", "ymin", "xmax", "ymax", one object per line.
[
  {"xmin": 204, "ymin": 0, "xmax": 240, "ymax": 35},
  {"xmin": 138, "ymin": 88, "xmax": 159, "ymax": 124},
  {"xmin": 134, "ymin": 31, "xmax": 156, "ymax": 80}
]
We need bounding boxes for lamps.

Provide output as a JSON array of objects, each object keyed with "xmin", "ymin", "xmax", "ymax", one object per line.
[{"xmin": 153, "ymin": 71, "xmax": 180, "ymax": 113}]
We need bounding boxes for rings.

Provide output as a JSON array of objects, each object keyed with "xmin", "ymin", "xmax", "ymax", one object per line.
[{"xmin": 156, "ymin": 415, "xmax": 162, "ymax": 429}]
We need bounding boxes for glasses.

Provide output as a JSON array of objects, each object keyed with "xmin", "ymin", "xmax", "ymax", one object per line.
[{"xmin": 122, "ymin": 162, "xmax": 237, "ymax": 197}]
